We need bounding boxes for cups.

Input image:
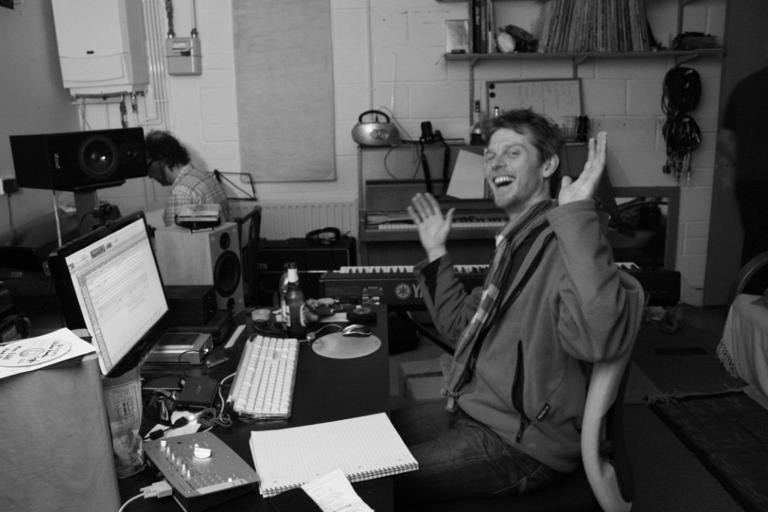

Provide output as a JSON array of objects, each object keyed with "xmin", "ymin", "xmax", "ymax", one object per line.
[{"xmin": 560, "ymin": 113, "xmax": 578, "ymax": 142}]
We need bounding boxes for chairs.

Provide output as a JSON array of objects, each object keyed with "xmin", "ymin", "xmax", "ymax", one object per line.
[
  {"xmin": 412, "ymin": 270, "xmax": 646, "ymax": 511},
  {"xmin": 234, "ymin": 204, "xmax": 263, "ymax": 256}
]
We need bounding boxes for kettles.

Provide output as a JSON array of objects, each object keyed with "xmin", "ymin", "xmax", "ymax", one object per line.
[{"xmin": 350, "ymin": 110, "xmax": 401, "ymax": 150}]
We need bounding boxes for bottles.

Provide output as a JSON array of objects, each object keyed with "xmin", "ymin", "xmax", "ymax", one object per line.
[
  {"xmin": 278, "ymin": 262, "xmax": 307, "ymax": 340},
  {"xmin": 577, "ymin": 112, "xmax": 590, "ymax": 144},
  {"xmin": 470, "ymin": 97, "xmax": 483, "ymax": 147}
]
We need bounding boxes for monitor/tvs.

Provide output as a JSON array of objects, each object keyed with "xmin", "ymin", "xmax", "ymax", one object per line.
[{"xmin": 47, "ymin": 209, "xmax": 174, "ymax": 380}]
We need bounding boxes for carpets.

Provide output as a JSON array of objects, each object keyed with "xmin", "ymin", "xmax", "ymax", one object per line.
[{"xmin": 646, "ymin": 387, "xmax": 766, "ymax": 510}]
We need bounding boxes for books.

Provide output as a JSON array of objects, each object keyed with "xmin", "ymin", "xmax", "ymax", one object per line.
[{"xmin": 246, "ymin": 410, "xmax": 423, "ymax": 498}]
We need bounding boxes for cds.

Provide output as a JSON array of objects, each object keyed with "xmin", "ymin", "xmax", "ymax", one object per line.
[{"xmin": 0, "ymin": 338, "xmax": 71, "ymax": 368}]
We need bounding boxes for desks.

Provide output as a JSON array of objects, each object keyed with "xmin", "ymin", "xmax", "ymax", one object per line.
[{"xmin": 119, "ymin": 303, "xmax": 397, "ymax": 512}]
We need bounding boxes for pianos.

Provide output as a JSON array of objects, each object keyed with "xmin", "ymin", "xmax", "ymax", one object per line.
[{"xmin": 322, "ymin": 262, "xmax": 652, "ymax": 308}]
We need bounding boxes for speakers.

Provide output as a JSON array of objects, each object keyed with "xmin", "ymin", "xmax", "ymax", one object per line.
[
  {"xmin": 243, "ymin": 237, "xmax": 357, "ymax": 309},
  {"xmin": 153, "ymin": 222, "xmax": 246, "ymax": 318},
  {"xmin": 9, "ymin": 126, "xmax": 149, "ymax": 194},
  {"xmin": 0, "ymin": 353, "xmax": 123, "ymax": 512}
]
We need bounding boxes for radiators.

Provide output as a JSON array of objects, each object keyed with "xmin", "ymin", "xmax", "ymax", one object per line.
[{"xmin": 229, "ymin": 198, "xmax": 360, "ymax": 265}]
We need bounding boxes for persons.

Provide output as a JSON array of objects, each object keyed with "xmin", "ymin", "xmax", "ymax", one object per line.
[
  {"xmin": 715, "ymin": 62, "xmax": 767, "ymax": 300},
  {"xmin": 382, "ymin": 105, "xmax": 630, "ymax": 507},
  {"xmin": 139, "ymin": 128, "xmax": 231, "ymax": 229}
]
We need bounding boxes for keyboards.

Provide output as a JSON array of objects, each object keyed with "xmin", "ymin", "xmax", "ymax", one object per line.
[{"xmin": 226, "ymin": 333, "xmax": 300, "ymax": 419}]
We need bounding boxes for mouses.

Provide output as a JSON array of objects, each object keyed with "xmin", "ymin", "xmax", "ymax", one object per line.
[{"xmin": 342, "ymin": 324, "xmax": 373, "ymax": 337}]
